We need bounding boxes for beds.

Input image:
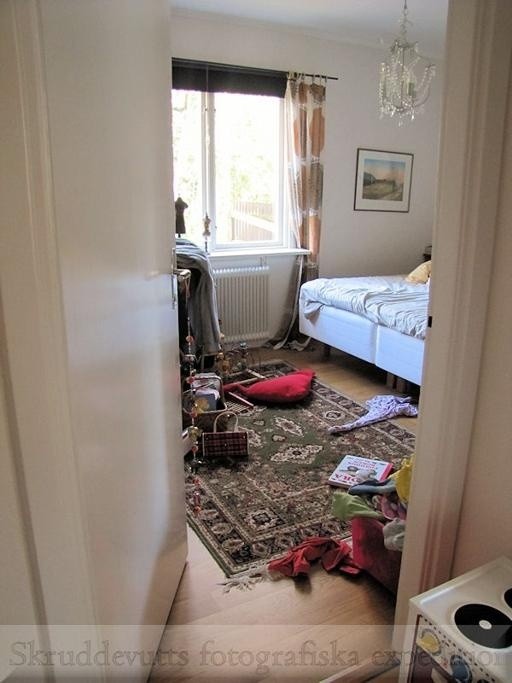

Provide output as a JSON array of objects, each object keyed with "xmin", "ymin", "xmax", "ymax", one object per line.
[{"xmin": 296, "ymin": 273, "xmax": 430, "ymax": 398}]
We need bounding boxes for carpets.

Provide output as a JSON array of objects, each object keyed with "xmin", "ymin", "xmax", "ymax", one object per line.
[{"xmin": 181, "ymin": 358, "xmax": 417, "ymax": 593}]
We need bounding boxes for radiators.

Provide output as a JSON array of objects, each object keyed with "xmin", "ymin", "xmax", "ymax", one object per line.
[{"xmin": 210, "ymin": 268, "xmax": 272, "ymax": 345}]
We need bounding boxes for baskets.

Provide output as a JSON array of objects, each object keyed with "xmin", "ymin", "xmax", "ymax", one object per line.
[{"xmin": 181, "ymin": 376, "xmax": 228, "ymax": 432}]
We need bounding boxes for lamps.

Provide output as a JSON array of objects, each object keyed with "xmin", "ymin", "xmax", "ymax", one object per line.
[{"xmin": 375, "ymin": 1, "xmax": 437, "ymax": 127}]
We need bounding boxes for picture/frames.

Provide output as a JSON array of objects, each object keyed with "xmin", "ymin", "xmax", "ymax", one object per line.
[{"xmin": 350, "ymin": 146, "xmax": 415, "ymax": 214}]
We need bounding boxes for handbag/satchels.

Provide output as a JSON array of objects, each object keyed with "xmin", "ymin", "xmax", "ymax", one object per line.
[{"xmin": 202, "ymin": 412, "xmax": 249, "ymax": 456}]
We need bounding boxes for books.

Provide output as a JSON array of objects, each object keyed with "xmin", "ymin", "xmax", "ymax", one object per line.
[{"xmin": 328, "ymin": 454, "xmax": 393, "ymax": 493}]
